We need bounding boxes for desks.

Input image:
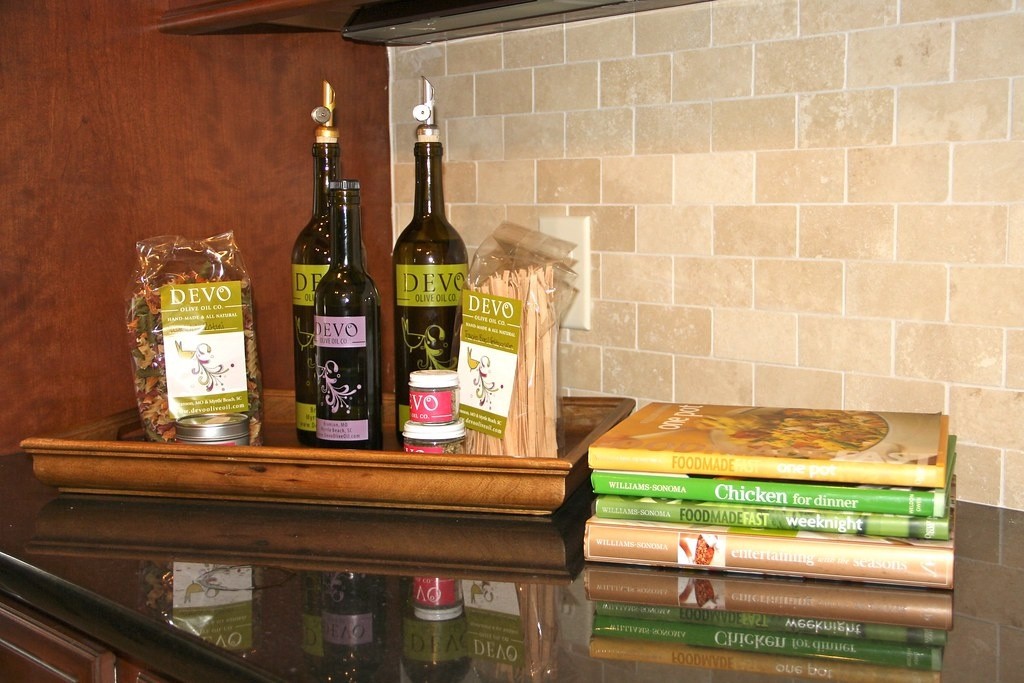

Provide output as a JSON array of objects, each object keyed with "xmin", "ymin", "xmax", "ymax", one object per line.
[{"xmin": 0, "ymin": 448, "xmax": 1024, "ymax": 682}]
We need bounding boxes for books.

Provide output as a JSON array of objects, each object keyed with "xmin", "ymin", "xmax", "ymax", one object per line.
[
  {"xmin": 589, "ymin": 635, "xmax": 941, "ymax": 683},
  {"xmin": 582, "ymin": 562, "xmax": 955, "ymax": 631},
  {"xmin": 588, "ymin": 401, "xmax": 950, "ymax": 490},
  {"xmin": 590, "ymin": 434, "xmax": 957, "ymax": 517},
  {"xmin": 596, "ymin": 482, "xmax": 953, "ymax": 540},
  {"xmin": 593, "ymin": 598, "xmax": 949, "ymax": 646},
  {"xmin": 583, "ymin": 474, "xmax": 957, "ymax": 590},
  {"xmin": 591, "ymin": 615, "xmax": 945, "ymax": 673}
]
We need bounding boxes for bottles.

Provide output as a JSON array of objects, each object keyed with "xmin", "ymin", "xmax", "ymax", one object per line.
[
  {"xmin": 299, "ymin": 570, "xmax": 388, "ymax": 683},
  {"xmin": 313, "ymin": 178, "xmax": 383, "ymax": 449},
  {"xmin": 392, "ymin": 76, "xmax": 469, "ymax": 453},
  {"xmin": 290, "ymin": 80, "xmax": 366, "ymax": 444},
  {"xmin": 400, "ymin": 575, "xmax": 471, "ymax": 683}
]
